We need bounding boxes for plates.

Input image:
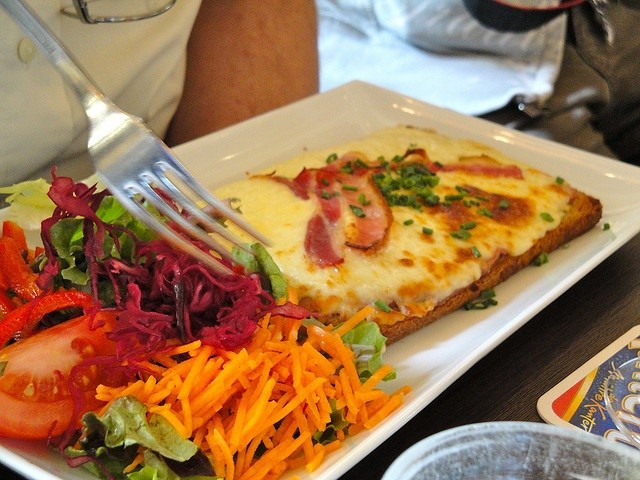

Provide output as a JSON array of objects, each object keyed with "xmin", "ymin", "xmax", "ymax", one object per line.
[
  {"xmin": 378, "ymin": 422, "xmax": 639, "ymax": 478},
  {"xmin": 0, "ymin": 77, "xmax": 639, "ymax": 480}
]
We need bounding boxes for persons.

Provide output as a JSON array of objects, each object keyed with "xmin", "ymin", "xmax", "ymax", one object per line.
[
  {"xmin": 311, "ymin": 0, "xmax": 640, "ymax": 172},
  {"xmin": 1, "ymin": 0, "xmax": 324, "ymax": 209}
]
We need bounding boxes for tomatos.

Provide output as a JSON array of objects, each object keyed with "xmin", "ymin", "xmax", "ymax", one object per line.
[{"xmin": 1, "ymin": 221, "xmax": 135, "ymax": 439}]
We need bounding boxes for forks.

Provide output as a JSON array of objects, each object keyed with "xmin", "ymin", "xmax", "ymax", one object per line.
[{"xmin": 0, "ymin": 0, "xmax": 274, "ymax": 283}]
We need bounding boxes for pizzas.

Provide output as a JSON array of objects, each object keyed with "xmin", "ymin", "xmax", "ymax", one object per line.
[{"xmin": 175, "ymin": 121, "xmax": 601, "ymax": 347}]
